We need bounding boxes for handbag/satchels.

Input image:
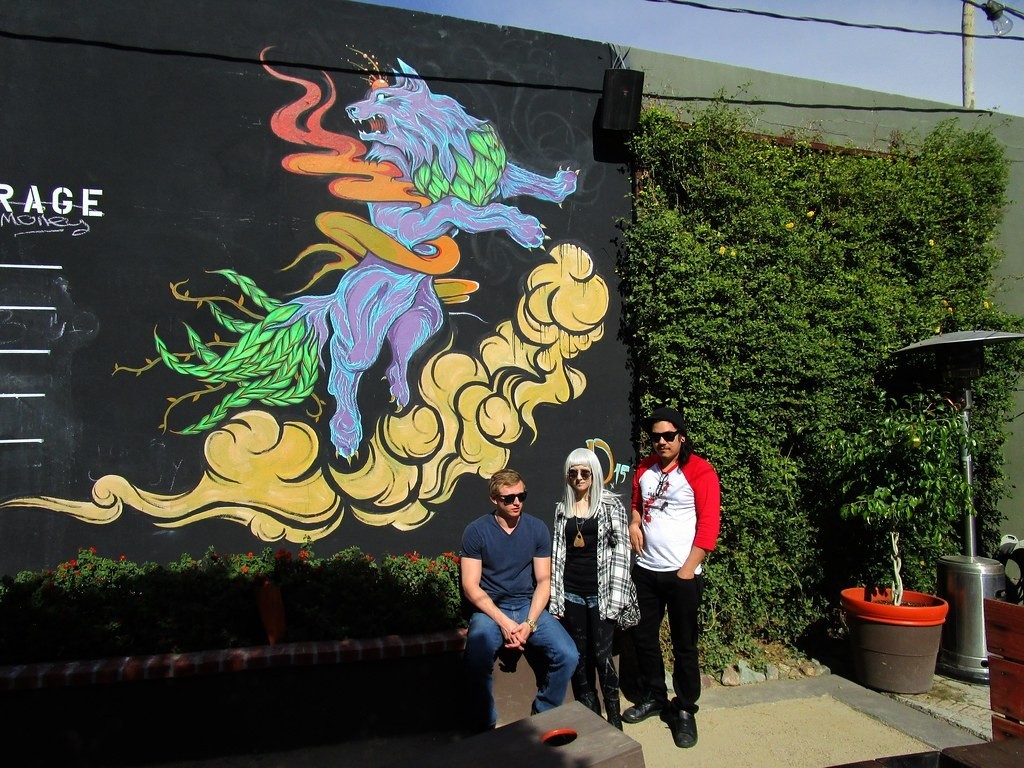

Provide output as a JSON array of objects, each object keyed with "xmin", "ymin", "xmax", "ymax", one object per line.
[{"xmin": 616, "ymin": 575, "xmax": 641, "ymax": 631}]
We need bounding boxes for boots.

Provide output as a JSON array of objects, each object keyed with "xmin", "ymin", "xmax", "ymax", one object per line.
[
  {"xmin": 579, "ymin": 689, "xmax": 600, "ymax": 716},
  {"xmin": 604, "ymin": 697, "xmax": 623, "ymax": 731}
]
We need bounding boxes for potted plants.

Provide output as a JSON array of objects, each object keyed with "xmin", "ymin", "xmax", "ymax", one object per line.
[{"xmin": 814, "ymin": 385, "xmax": 988, "ymax": 697}]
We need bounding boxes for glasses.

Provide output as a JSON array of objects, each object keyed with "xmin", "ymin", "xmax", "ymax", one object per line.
[
  {"xmin": 568, "ymin": 470, "xmax": 592, "ymax": 480},
  {"xmin": 650, "ymin": 430, "xmax": 679, "ymax": 442},
  {"xmin": 656, "ymin": 473, "xmax": 668, "ymax": 496},
  {"xmin": 496, "ymin": 490, "xmax": 528, "ymax": 504}
]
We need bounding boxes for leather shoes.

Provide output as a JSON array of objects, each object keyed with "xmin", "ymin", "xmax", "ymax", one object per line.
[
  {"xmin": 623, "ymin": 699, "xmax": 669, "ymax": 723},
  {"xmin": 674, "ymin": 709, "xmax": 698, "ymax": 747}
]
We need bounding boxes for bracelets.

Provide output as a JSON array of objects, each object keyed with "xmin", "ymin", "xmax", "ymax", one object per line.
[{"xmin": 525, "ymin": 619, "xmax": 538, "ymax": 633}]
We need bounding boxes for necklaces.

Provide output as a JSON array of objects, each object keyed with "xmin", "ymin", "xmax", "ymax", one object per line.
[{"xmin": 574, "ymin": 498, "xmax": 585, "ymax": 548}]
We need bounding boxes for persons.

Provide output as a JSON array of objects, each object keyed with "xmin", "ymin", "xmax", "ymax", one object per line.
[
  {"xmin": 460, "ymin": 469, "xmax": 580, "ymax": 735},
  {"xmin": 547, "ymin": 448, "xmax": 632, "ymax": 732},
  {"xmin": 627, "ymin": 408, "xmax": 720, "ymax": 748}
]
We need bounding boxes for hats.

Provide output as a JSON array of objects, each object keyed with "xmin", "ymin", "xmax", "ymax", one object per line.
[{"xmin": 639, "ymin": 408, "xmax": 687, "ymax": 435}]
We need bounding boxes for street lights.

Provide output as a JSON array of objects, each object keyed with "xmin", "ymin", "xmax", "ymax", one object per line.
[{"xmin": 962, "ymin": 0, "xmax": 1013, "ymax": 110}]
